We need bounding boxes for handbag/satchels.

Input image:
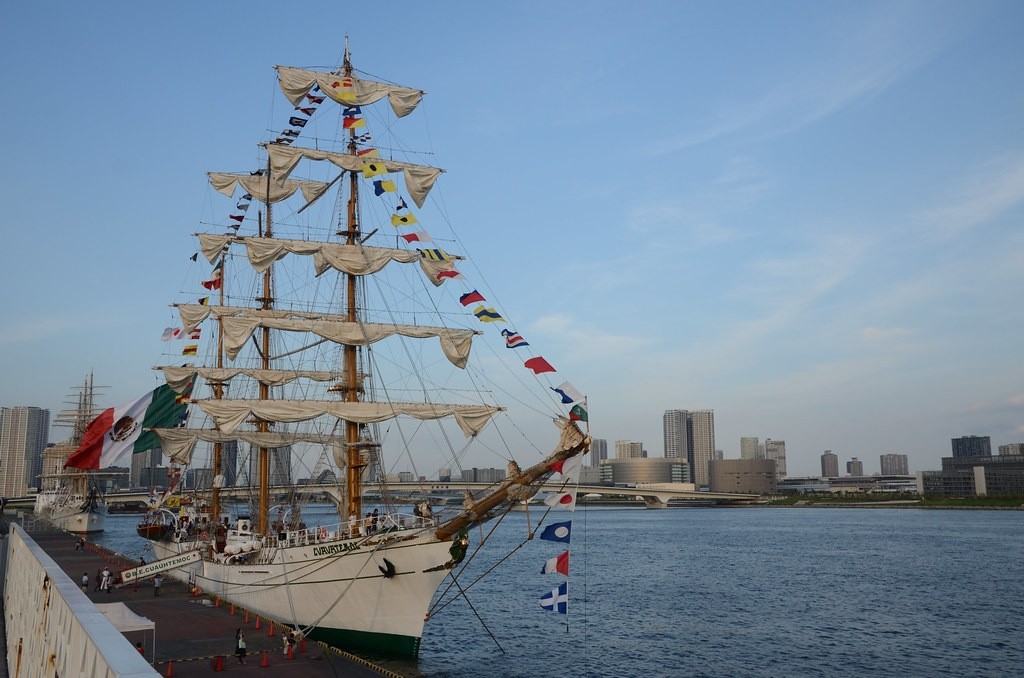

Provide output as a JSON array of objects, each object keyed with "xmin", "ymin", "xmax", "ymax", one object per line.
[{"xmin": 239, "ymin": 639, "xmax": 246, "ymax": 648}]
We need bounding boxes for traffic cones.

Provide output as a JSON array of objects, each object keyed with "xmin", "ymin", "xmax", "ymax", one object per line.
[
  {"xmin": 286, "ymin": 642, "xmax": 295, "ymax": 660},
  {"xmin": 165, "ymin": 656, "xmax": 175, "ymax": 678},
  {"xmin": 268, "ymin": 624, "xmax": 275, "ymax": 636},
  {"xmin": 299, "ymin": 639, "xmax": 306, "ymax": 653},
  {"xmin": 260, "ymin": 651, "xmax": 270, "ymax": 668},
  {"xmin": 254, "ymin": 616, "xmax": 260, "ymax": 629},
  {"xmin": 213, "ymin": 654, "xmax": 225, "ymax": 672},
  {"xmin": 243, "ymin": 608, "xmax": 250, "ymax": 624},
  {"xmin": 229, "ymin": 604, "xmax": 236, "ymax": 617},
  {"xmin": 214, "ymin": 596, "xmax": 221, "ymax": 608},
  {"xmin": 194, "ymin": 586, "xmax": 200, "ymax": 597}
]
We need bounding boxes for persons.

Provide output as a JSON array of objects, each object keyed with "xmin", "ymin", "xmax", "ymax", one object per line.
[
  {"xmin": 76, "ymin": 540, "xmax": 84, "ymax": 551},
  {"xmin": 235, "ymin": 629, "xmax": 246, "ymax": 664},
  {"xmin": 414, "ymin": 503, "xmax": 432, "ymax": 525},
  {"xmin": 154, "ymin": 574, "xmax": 164, "ymax": 596},
  {"xmin": 168, "ymin": 520, "xmax": 230, "ymax": 537},
  {"xmin": 282, "ymin": 633, "xmax": 297, "ymax": 657},
  {"xmin": 366, "ymin": 508, "xmax": 378, "ymax": 534},
  {"xmin": 140, "ymin": 557, "xmax": 146, "ymax": 566},
  {"xmin": 82, "ymin": 567, "xmax": 114, "ymax": 594}
]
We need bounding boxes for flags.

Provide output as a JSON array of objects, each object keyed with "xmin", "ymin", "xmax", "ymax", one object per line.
[
  {"xmin": 540, "ymin": 582, "xmax": 568, "ymax": 614},
  {"xmin": 551, "ymin": 382, "xmax": 586, "ymax": 404},
  {"xmin": 549, "ymin": 455, "xmax": 583, "ymax": 482},
  {"xmin": 540, "ymin": 550, "xmax": 569, "ymax": 576},
  {"xmin": 540, "ymin": 520, "xmax": 571, "ymax": 544},
  {"xmin": 332, "ymin": 78, "xmax": 556, "ymax": 375},
  {"xmin": 246, "ymin": 95, "xmax": 327, "ymax": 176},
  {"xmin": 63, "ymin": 374, "xmax": 197, "ymax": 468},
  {"xmin": 161, "ymin": 195, "xmax": 252, "ymax": 488},
  {"xmin": 544, "ymin": 489, "xmax": 577, "ymax": 510},
  {"xmin": 569, "ymin": 401, "xmax": 588, "ymax": 422}
]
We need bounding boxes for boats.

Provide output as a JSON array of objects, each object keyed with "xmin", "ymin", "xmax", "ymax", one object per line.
[
  {"xmin": 136, "ymin": 523, "xmax": 162, "ymax": 542},
  {"xmin": 271, "ymin": 520, "xmax": 310, "ymax": 536}
]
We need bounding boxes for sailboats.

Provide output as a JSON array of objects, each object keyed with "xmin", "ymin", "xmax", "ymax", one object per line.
[
  {"xmin": 145, "ymin": 31, "xmax": 595, "ymax": 667},
  {"xmin": 32, "ymin": 366, "xmax": 132, "ymax": 536}
]
